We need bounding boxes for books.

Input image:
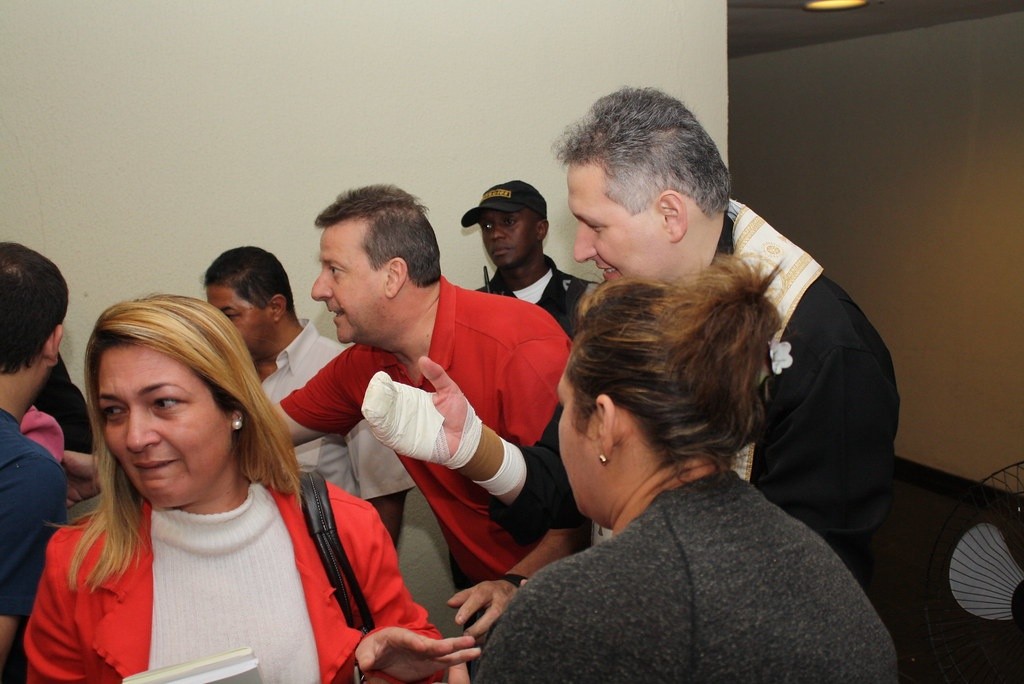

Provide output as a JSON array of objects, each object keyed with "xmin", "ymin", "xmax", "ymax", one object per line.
[{"xmin": 122, "ymin": 646, "xmax": 263, "ymax": 684}]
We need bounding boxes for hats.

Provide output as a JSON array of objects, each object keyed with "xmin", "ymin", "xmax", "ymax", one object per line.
[{"xmin": 461, "ymin": 180, "xmax": 547, "ymax": 228}]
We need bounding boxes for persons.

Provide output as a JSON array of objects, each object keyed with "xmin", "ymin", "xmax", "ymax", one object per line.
[
  {"xmin": 461, "ymin": 179, "xmax": 601, "ymax": 339},
  {"xmin": 205, "ymin": 245, "xmax": 417, "ymax": 547},
  {"xmin": 0, "ymin": 242, "xmax": 95, "ymax": 670},
  {"xmin": 66, "ymin": 183, "xmax": 574, "ymax": 638},
  {"xmin": 467, "ymin": 256, "xmax": 898, "ymax": 684},
  {"xmin": 362, "ymin": 88, "xmax": 901, "ymax": 586},
  {"xmin": 23, "ymin": 293, "xmax": 481, "ymax": 684}
]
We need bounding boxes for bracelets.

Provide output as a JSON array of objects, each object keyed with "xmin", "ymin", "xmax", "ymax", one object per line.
[{"xmin": 502, "ymin": 573, "xmax": 527, "ymax": 588}]
